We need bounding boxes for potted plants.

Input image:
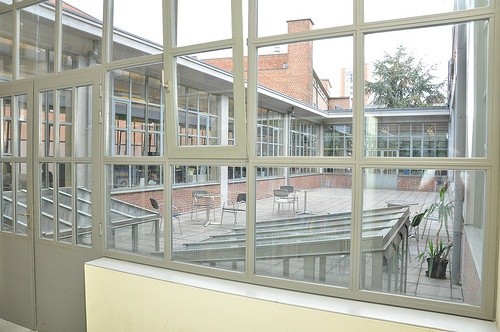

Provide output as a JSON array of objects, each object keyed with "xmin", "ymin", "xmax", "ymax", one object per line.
[{"xmin": 409, "ymin": 182, "xmax": 456, "ymax": 279}]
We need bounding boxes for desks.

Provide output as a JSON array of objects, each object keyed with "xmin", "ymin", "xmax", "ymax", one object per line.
[
  {"xmin": 294, "ymin": 188, "xmax": 316, "ymax": 216},
  {"xmin": 193, "ymin": 195, "xmax": 222, "ymax": 228},
  {"xmin": 386, "ymin": 199, "xmax": 416, "ymax": 208}
]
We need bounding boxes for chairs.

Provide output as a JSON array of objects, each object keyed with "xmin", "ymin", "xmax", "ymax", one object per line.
[
  {"xmin": 220, "ymin": 193, "xmax": 246, "ymax": 228},
  {"xmin": 150, "ymin": 198, "xmax": 183, "ymax": 235},
  {"xmin": 273, "ymin": 186, "xmax": 299, "ymax": 215},
  {"xmin": 189, "ymin": 191, "xmax": 215, "ymax": 225},
  {"xmin": 406, "ymin": 206, "xmax": 442, "ymax": 264}
]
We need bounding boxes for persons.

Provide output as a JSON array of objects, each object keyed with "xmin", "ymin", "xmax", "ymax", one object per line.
[
  {"xmin": 3, "ymin": 163, "xmax": 12, "ymax": 190},
  {"xmin": 42, "ymin": 164, "xmax": 53, "ymax": 188}
]
